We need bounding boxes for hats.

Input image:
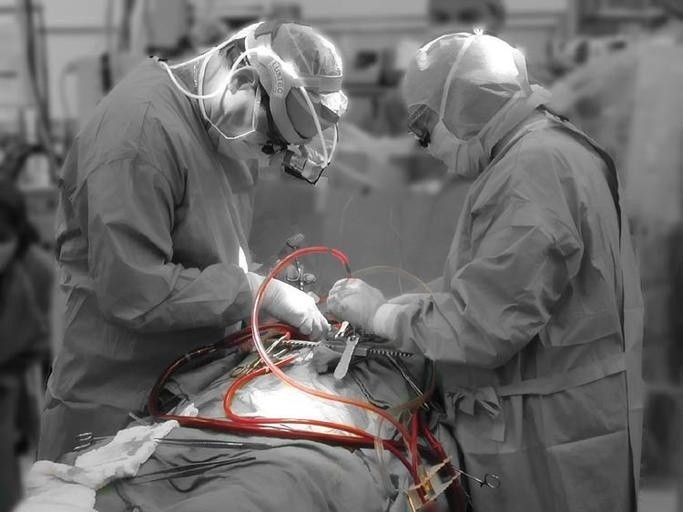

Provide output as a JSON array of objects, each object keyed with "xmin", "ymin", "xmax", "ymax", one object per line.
[
  {"xmin": 245, "ymin": 21, "xmax": 344, "ymax": 145},
  {"xmin": 404, "ymin": 32, "xmax": 526, "ymax": 139}
]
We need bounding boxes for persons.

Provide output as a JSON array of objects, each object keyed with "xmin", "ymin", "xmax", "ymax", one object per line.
[
  {"xmin": 35, "ymin": 20, "xmax": 346, "ymax": 463},
  {"xmin": 328, "ymin": 31, "xmax": 646, "ymax": 510},
  {"xmin": 50, "ymin": 325, "xmax": 435, "ymax": 510}
]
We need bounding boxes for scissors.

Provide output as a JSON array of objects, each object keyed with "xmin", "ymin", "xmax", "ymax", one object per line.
[
  {"xmin": 454, "ymin": 467, "xmax": 501, "ymax": 512},
  {"xmin": 231, "ymin": 336, "xmax": 287, "ymax": 378},
  {"xmin": 72, "ymin": 432, "xmax": 112, "ymax": 451}
]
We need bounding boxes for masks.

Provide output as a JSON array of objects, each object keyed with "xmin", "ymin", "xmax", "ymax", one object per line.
[
  {"xmin": 217, "ymin": 124, "xmax": 280, "ymax": 162},
  {"xmin": 428, "ymin": 122, "xmax": 488, "ymax": 176}
]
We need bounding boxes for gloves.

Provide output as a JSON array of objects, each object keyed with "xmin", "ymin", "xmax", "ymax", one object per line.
[
  {"xmin": 245, "ymin": 270, "xmax": 331, "ymax": 341},
  {"xmin": 327, "ymin": 278, "xmax": 402, "ymax": 339}
]
